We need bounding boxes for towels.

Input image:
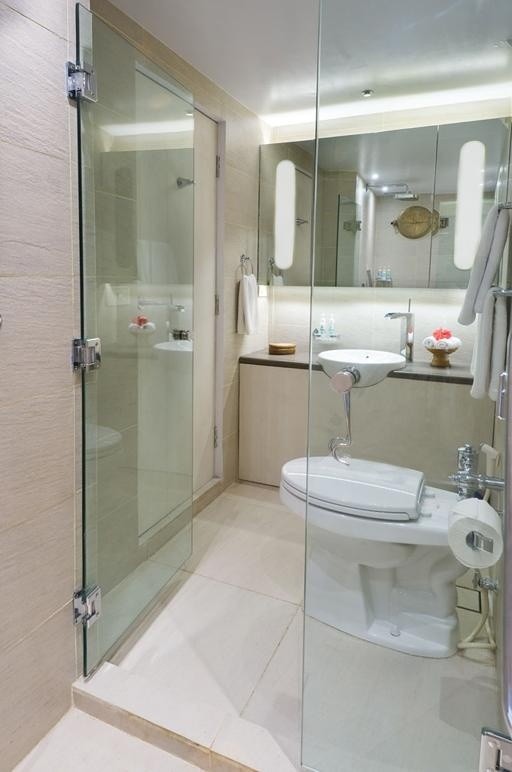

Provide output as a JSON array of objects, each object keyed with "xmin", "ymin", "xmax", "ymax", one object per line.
[
  {"xmin": 423, "ymin": 335, "xmax": 461, "ymax": 350},
  {"xmin": 457, "ymin": 200, "xmax": 508, "ymax": 402},
  {"xmin": 236, "ymin": 275, "xmax": 256, "ymax": 337}
]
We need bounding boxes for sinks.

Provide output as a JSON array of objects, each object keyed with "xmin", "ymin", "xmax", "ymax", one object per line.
[
  {"xmin": 318, "ymin": 348, "xmax": 406, "ymax": 389},
  {"xmin": 153, "ymin": 340, "xmax": 193, "ymax": 364}
]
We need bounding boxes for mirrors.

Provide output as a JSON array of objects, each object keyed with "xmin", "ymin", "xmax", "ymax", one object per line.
[{"xmin": 255, "ymin": 116, "xmax": 511, "ymax": 293}]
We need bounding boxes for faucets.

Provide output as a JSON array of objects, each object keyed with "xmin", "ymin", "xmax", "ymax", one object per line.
[{"xmin": 384, "ymin": 311, "xmax": 414, "ymax": 362}]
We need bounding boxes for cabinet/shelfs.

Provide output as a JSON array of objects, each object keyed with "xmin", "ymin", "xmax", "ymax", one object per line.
[{"xmin": 236, "ymin": 362, "xmax": 494, "ymax": 503}]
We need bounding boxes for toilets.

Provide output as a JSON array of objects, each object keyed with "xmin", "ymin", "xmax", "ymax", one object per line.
[
  {"xmin": 278, "ymin": 456, "xmax": 474, "ymax": 658},
  {"xmin": 84, "ymin": 424, "xmax": 128, "ymax": 488}
]
